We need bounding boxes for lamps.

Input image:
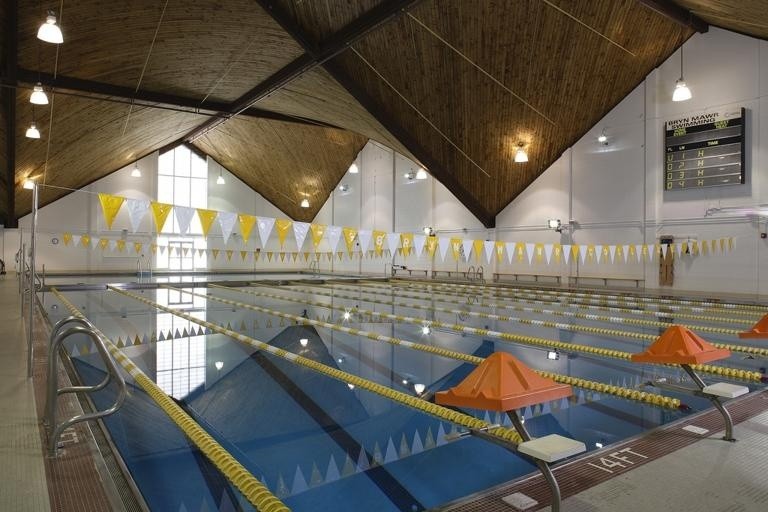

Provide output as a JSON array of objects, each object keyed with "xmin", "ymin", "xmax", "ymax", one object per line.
[
  {"xmin": 598, "ymin": 125, "xmax": 610, "ymax": 142},
  {"xmin": 514, "ymin": 141, "xmax": 529, "ymax": 162},
  {"xmin": 545, "ymin": 346, "xmax": 561, "ymax": 361},
  {"xmin": 301, "ymin": 186, "xmax": 310, "ymax": 207},
  {"xmin": 669, "ymin": 26, "xmax": 694, "ymax": 101},
  {"xmin": 214, "ymin": 361, "xmax": 224, "ymax": 379},
  {"xmin": 130, "ymin": 150, "xmax": 143, "ymax": 177},
  {"xmin": 548, "ymin": 219, "xmax": 564, "ymax": 233},
  {"xmin": 300, "ymin": 338, "xmax": 309, "ymax": 355},
  {"xmin": 20, "ymin": 1, "xmax": 64, "ymax": 191},
  {"xmin": 422, "ymin": 227, "xmax": 436, "ymax": 239},
  {"xmin": 216, "ymin": 162, "xmax": 225, "ymax": 185}
]
